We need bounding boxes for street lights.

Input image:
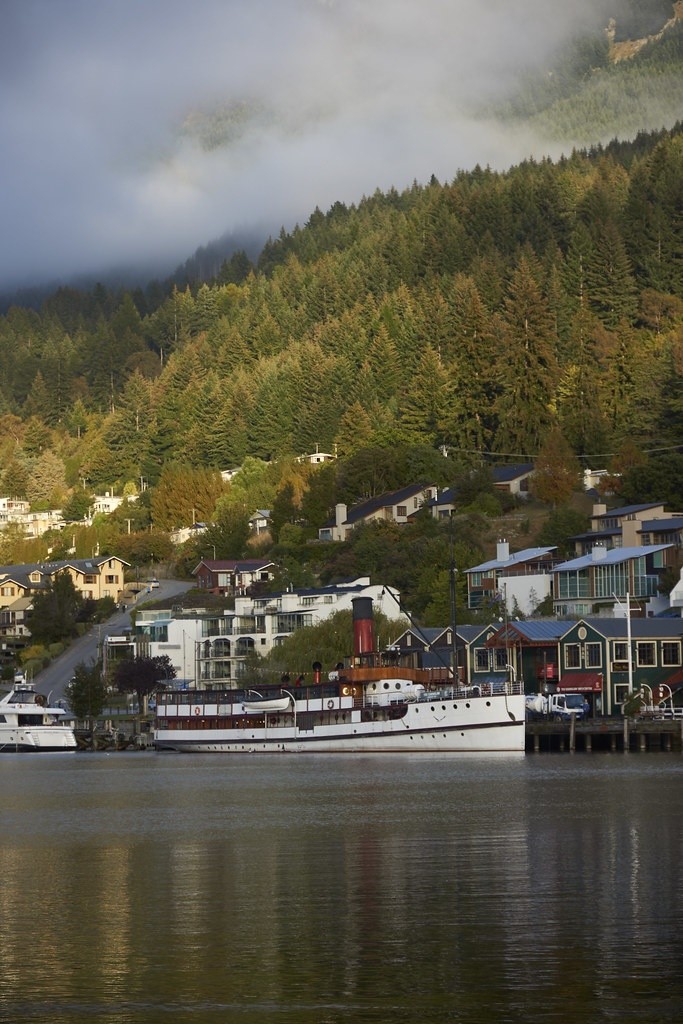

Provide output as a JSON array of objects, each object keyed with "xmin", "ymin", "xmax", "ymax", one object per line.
[{"xmin": 207, "ymin": 544, "xmax": 216, "ymax": 561}]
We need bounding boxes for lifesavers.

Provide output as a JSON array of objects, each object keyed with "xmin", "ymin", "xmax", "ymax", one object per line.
[
  {"xmin": 328, "ymin": 700, "xmax": 334, "ymax": 709},
  {"xmin": 195, "ymin": 707, "xmax": 200, "ymax": 715}
]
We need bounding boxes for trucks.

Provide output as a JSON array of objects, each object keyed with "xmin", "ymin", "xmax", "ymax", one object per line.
[{"xmin": 526, "ymin": 692, "xmax": 587, "ymax": 720}]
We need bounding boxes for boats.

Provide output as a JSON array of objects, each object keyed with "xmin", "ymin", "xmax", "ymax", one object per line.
[
  {"xmin": 152, "ymin": 509, "xmax": 526, "ymax": 752},
  {"xmin": 0, "ymin": 666, "xmax": 79, "ymax": 752}
]
